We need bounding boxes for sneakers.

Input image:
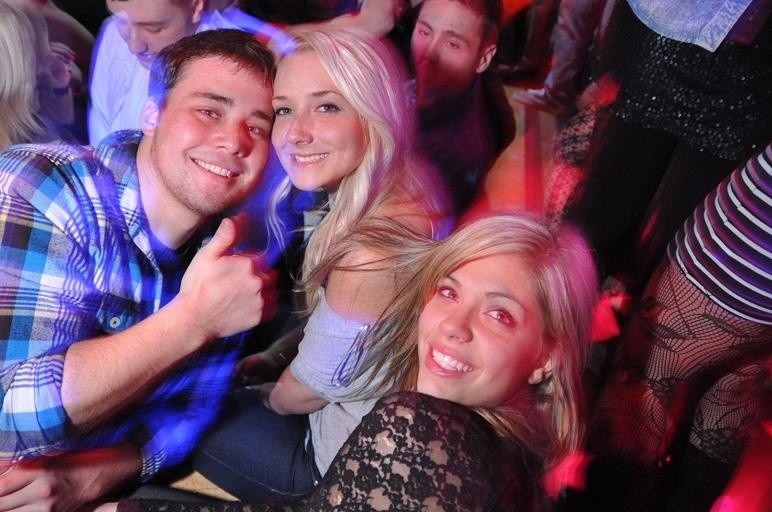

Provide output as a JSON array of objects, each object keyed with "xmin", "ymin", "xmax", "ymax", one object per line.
[{"xmin": 512, "ymin": 89, "xmax": 568, "ymax": 118}]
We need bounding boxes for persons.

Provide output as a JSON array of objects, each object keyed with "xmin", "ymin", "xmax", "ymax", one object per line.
[{"xmin": 3, "ymin": 0, "xmax": 772, "ymax": 511}]
